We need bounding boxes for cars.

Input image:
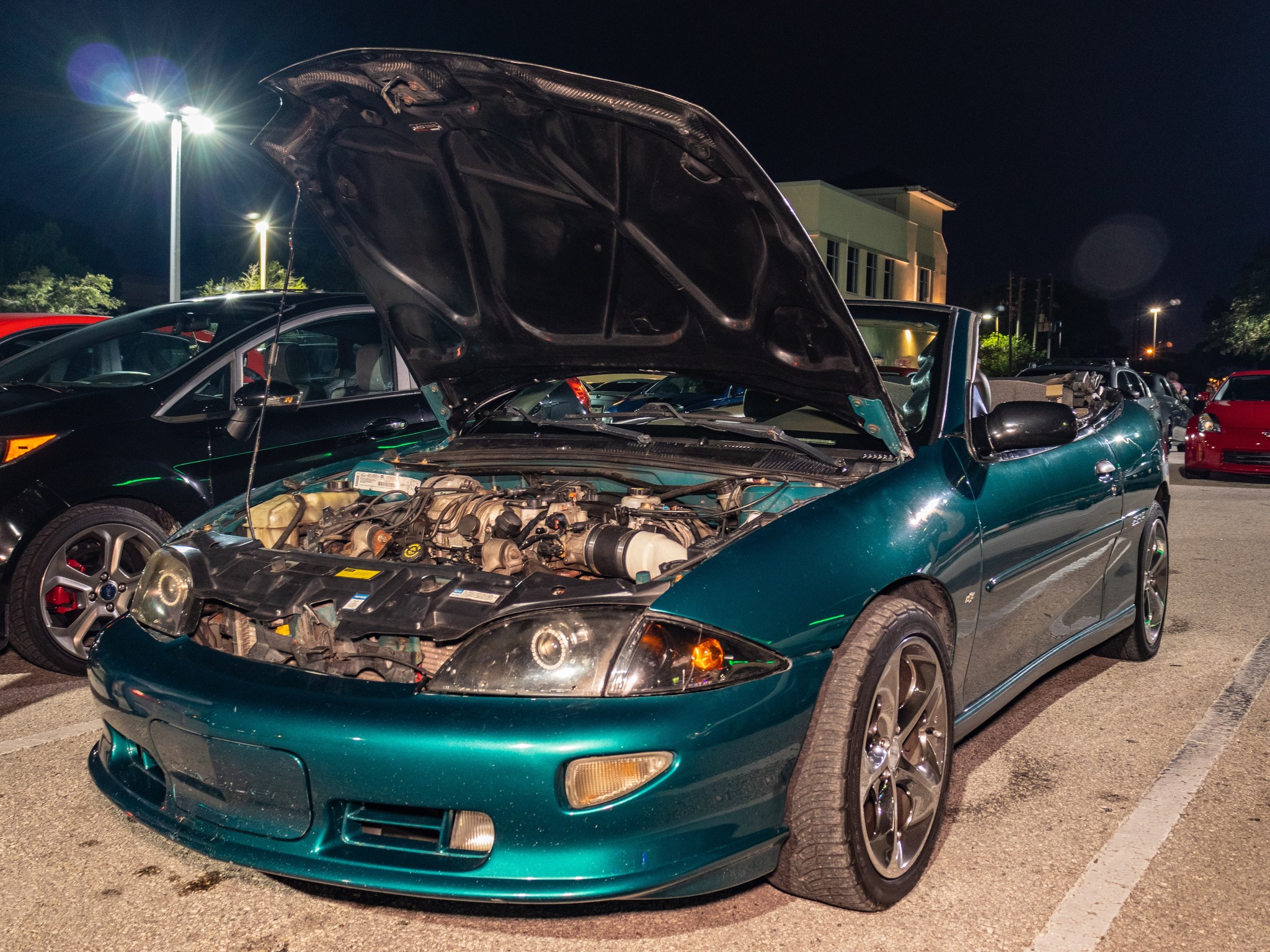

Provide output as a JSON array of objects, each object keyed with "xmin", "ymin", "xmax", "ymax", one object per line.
[
  {"xmin": 1184, "ymin": 371, "xmax": 1270, "ymax": 480},
  {"xmin": 1018, "ymin": 357, "xmax": 1196, "ymax": 454},
  {"xmin": 0, "ymin": 288, "xmax": 438, "ymax": 679},
  {"xmin": 0, "ymin": 311, "xmax": 266, "ymax": 384}
]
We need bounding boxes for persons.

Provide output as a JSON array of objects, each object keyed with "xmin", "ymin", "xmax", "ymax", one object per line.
[
  {"xmin": 1166, "ymin": 371, "xmax": 1187, "ymax": 396},
  {"xmin": 874, "ymin": 352, "xmax": 884, "ymax": 366}
]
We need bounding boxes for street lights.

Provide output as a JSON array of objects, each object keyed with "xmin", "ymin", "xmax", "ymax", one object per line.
[
  {"xmin": 1150, "ymin": 308, "xmax": 1161, "ymax": 359},
  {"xmin": 255, "ymin": 221, "xmax": 271, "ymax": 290},
  {"xmin": 982, "ymin": 314, "xmax": 999, "ymax": 346},
  {"xmin": 126, "ymin": 93, "xmax": 215, "ymax": 303}
]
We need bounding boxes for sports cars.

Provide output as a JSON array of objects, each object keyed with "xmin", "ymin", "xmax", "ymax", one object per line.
[{"xmin": 83, "ymin": 45, "xmax": 1172, "ymax": 914}]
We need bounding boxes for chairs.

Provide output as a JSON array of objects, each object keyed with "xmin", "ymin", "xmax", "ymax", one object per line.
[
  {"xmin": 328, "ymin": 341, "xmax": 396, "ymax": 401},
  {"xmin": 982, "ymin": 377, "xmax": 1076, "ymax": 413},
  {"xmin": 260, "ymin": 338, "xmax": 325, "ymax": 399}
]
